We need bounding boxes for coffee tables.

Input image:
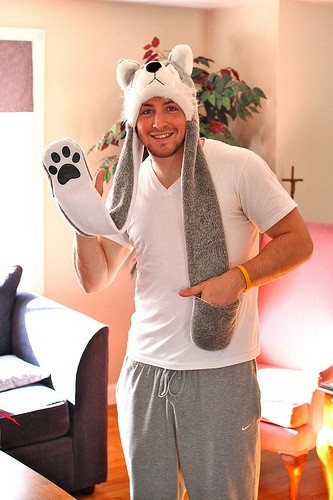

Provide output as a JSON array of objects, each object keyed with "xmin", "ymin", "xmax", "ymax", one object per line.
[{"xmin": 0, "ymin": 450, "xmax": 77, "ymax": 500}]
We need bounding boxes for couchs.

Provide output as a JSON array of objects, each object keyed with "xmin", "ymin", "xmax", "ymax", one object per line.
[{"xmin": 0, "ymin": 291, "xmax": 110, "ymax": 496}]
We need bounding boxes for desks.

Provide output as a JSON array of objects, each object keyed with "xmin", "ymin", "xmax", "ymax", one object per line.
[{"xmin": 311, "ymin": 387, "xmax": 333, "ymax": 500}]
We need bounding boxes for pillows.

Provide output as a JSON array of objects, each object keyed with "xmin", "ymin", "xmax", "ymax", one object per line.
[
  {"xmin": 0, "ymin": 355, "xmax": 52, "ymax": 392},
  {"xmin": 0, "ymin": 264, "xmax": 23, "ymax": 357}
]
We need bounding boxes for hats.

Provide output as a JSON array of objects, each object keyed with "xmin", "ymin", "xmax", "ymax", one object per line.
[{"xmin": 117, "ymin": 45, "xmax": 199, "ymax": 127}]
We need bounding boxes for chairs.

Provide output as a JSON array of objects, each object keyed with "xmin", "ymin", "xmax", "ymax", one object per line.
[{"xmin": 180, "ymin": 222, "xmax": 333, "ymax": 500}]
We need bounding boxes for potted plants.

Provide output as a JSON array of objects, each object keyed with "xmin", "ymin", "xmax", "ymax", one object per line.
[{"xmin": 87, "ymin": 38, "xmax": 266, "ymax": 184}]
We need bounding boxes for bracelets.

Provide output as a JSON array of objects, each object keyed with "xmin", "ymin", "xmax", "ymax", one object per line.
[{"xmin": 236, "ymin": 265, "xmax": 251, "ymax": 293}]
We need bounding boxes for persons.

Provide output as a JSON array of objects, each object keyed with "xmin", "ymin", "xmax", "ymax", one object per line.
[{"xmin": 43, "ymin": 61, "xmax": 314, "ymax": 500}]
